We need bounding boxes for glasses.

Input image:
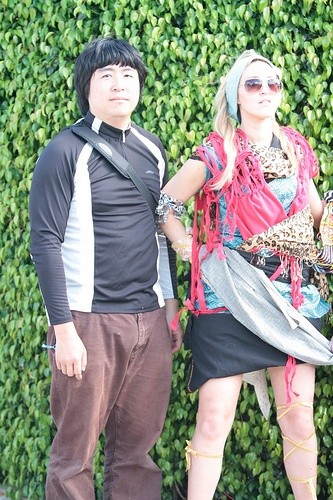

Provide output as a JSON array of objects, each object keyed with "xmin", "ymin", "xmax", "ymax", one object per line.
[{"xmin": 238, "ymin": 77, "xmax": 285, "ymax": 94}]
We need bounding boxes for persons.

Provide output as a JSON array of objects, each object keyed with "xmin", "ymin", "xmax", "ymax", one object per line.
[
  {"xmin": 21, "ymin": 36, "xmax": 187, "ymax": 499},
  {"xmin": 153, "ymin": 49, "xmax": 333, "ymax": 500}
]
